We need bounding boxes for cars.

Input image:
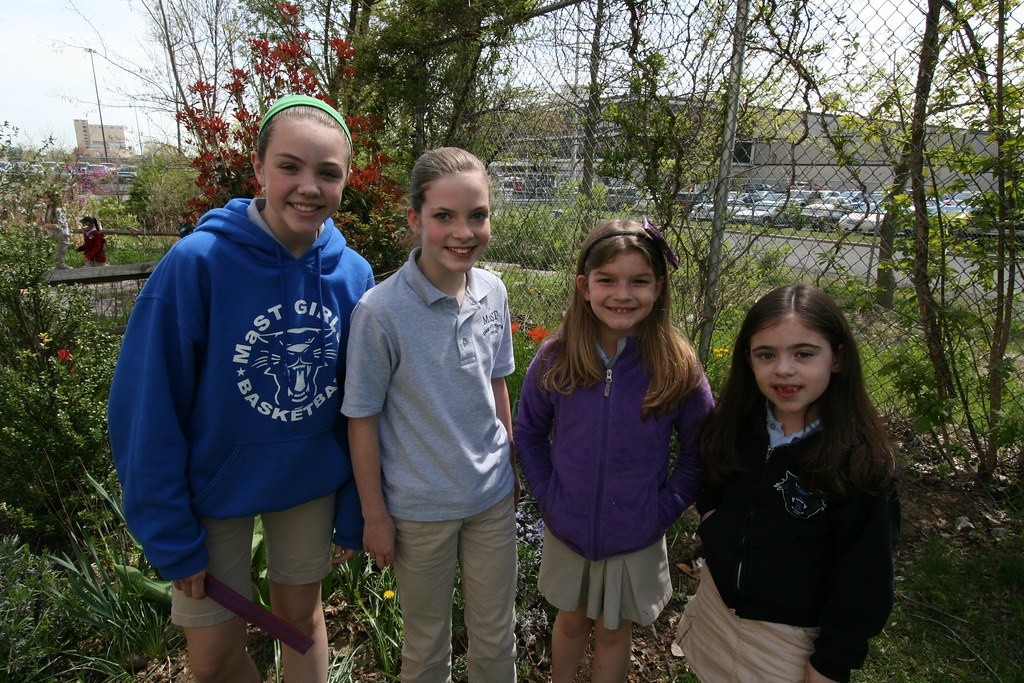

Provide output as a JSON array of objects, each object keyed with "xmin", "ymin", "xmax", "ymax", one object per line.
[
  {"xmin": 691, "ymin": 187, "xmax": 1023, "ymax": 229},
  {"xmin": 509, "ymin": 178, "xmax": 562, "ymax": 196},
  {"xmin": 1, "ymin": 162, "xmax": 145, "ymax": 183}
]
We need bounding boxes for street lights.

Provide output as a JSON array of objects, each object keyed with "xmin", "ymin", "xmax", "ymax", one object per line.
[{"xmin": 84, "ymin": 48, "xmax": 108, "ymax": 162}]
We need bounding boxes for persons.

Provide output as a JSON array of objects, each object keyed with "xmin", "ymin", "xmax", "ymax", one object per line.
[
  {"xmin": 33, "ymin": 191, "xmax": 73, "ymax": 269},
  {"xmin": 74, "ymin": 216, "xmax": 110, "ymax": 266},
  {"xmin": 177, "ymin": 213, "xmax": 196, "ymax": 239},
  {"xmin": 107, "ymin": 94, "xmax": 378, "ymax": 683},
  {"xmin": 338, "ymin": 144, "xmax": 519, "ymax": 683},
  {"xmin": 515, "ymin": 218, "xmax": 715, "ymax": 683},
  {"xmin": 676, "ymin": 282, "xmax": 901, "ymax": 683}
]
